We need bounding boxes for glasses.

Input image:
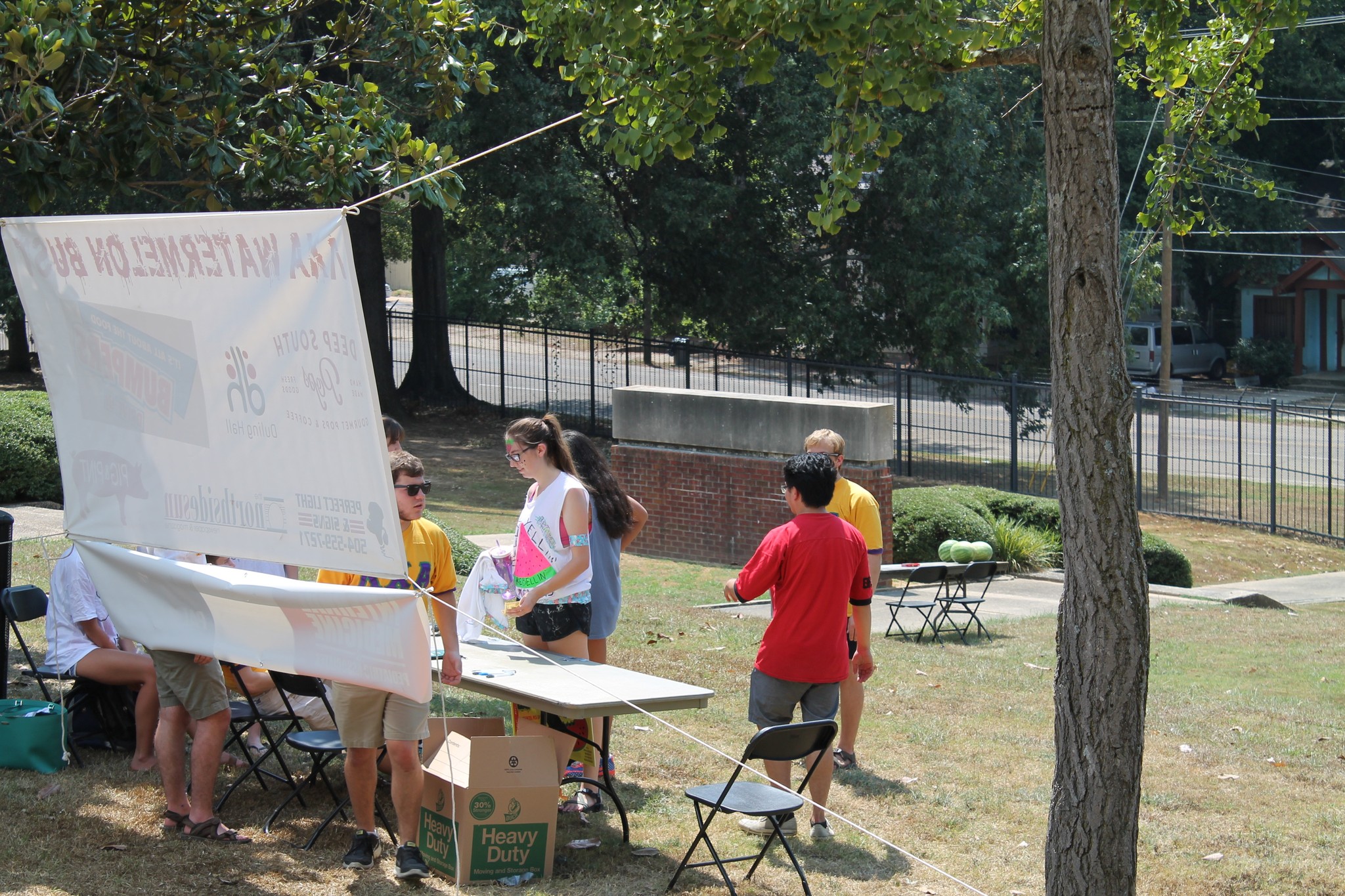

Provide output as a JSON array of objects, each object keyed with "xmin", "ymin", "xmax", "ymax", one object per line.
[
  {"xmin": 505, "ymin": 443, "xmax": 538, "ymax": 462},
  {"xmin": 822, "ymin": 453, "xmax": 839, "ymax": 457},
  {"xmin": 781, "ymin": 485, "xmax": 793, "ymax": 494},
  {"xmin": 394, "ymin": 481, "xmax": 432, "ymax": 496}
]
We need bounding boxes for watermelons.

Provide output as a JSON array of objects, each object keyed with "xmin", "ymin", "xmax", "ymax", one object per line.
[
  {"xmin": 971, "ymin": 541, "xmax": 992, "ymax": 561},
  {"xmin": 938, "ymin": 539, "xmax": 958, "ymax": 562},
  {"xmin": 950, "ymin": 540, "xmax": 975, "ymax": 563}
]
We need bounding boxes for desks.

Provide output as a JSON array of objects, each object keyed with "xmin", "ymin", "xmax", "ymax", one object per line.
[
  {"xmin": 876, "ymin": 559, "xmax": 1009, "ymax": 644},
  {"xmin": 429, "ymin": 630, "xmax": 715, "ymax": 864}
]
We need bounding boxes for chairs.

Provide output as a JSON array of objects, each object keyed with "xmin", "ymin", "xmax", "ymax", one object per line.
[
  {"xmin": 884, "ymin": 561, "xmax": 993, "ymax": 646},
  {"xmin": 662, "ymin": 720, "xmax": 838, "ymax": 896},
  {"xmin": 0, "ymin": 584, "xmax": 121, "ymax": 765},
  {"xmin": 187, "ymin": 659, "xmax": 304, "ymax": 815},
  {"xmin": 264, "ymin": 668, "xmax": 397, "ymax": 851}
]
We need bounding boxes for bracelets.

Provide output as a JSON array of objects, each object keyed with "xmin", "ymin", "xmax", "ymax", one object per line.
[{"xmin": 568, "ymin": 533, "xmax": 590, "ymax": 548}]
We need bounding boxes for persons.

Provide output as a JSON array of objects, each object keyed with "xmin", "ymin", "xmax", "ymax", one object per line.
[
  {"xmin": 561, "ymin": 429, "xmax": 648, "ymax": 778},
  {"xmin": 457, "ymin": 412, "xmax": 605, "ymax": 813},
  {"xmin": 46, "ymin": 414, "xmax": 462, "ymax": 879},
  {"xmin": 803, "ymin": 428, "xmax": 883, "ymax": 773},
  {"xmin": 724, "ymin": 452, "xmax": 875, "ymax": 841}
]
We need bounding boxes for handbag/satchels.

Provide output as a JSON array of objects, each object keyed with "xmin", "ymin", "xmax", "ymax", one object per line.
[
  {"xmin": 54, "ymin": 677, "xmax": 140, "ymax": 752},
  {"xmin": 0, "ymin": 698, "xmax": 68, "ymax": 775}
]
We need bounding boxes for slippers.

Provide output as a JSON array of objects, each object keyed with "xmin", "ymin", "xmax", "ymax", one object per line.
[
  {"xmin": 245, "ymin": 746, "xmax": 268, "ymax": 758},
  {"xmin": 218, "ymin": 753, "xmax": 251, "ymax": 773}
]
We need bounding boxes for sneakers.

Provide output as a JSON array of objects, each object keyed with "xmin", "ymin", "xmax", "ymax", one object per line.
[
  {"xmin": 809, "ymin": 817, "xmax": 835, "ymax": 841},
  {"xmin": 738, "ymin": 812, "xmax": 797, "ymax": 836},
  {"xmin": 562, "ymin": 754, "xmax": 615, "ymax": 778},
  {"xmin": 341, "ymin": 829, "xmax": 382, "ymax": 869},
  {"xmin": 393, "ymin": 841, "xmax": 431, "ymax": 879}
]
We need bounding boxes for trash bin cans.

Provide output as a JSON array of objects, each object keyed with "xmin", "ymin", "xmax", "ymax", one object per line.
[{"xmin": 673, "ymin": 338, "xmax": 690, "ymax": 365}]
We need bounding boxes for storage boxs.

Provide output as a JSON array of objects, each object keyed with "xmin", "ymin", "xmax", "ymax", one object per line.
[{"xmin": 422, "ymin": 715, "xmax": 577, "ymax": 887}]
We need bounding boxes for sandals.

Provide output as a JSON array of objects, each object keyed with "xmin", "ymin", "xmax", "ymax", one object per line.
[
  {"xmin": 558, "ymin": 788, "xmax": 604, "ymax": 814},
  {"xmin": 159, "ymin": 809, "xmax": 190, "ymax": 831},
  {"xmin": 832, "ymin": 747, "xmax": 857, "ymax": 776},
  {"xmin": 179, "ymin": 816, "xmax": 252, "ymax": 844}
]
